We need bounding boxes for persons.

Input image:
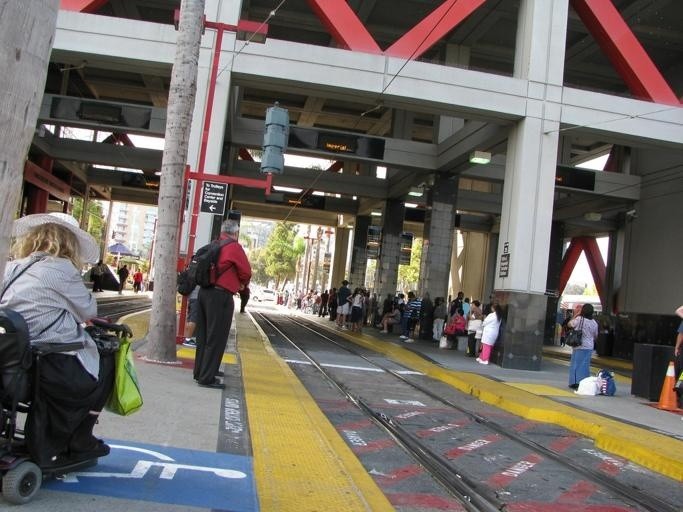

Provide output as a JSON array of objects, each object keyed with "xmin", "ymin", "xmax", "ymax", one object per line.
[
  {"xmin": 91, "ymin": 263, "xmax": 103, "ymax": 292},
  {"xmin": 566, "ymin": 302, "xmax": 600, "ymax": 389},
  {"xmin": 238, "ymin": 283, "xmax": 251, "ymax": 313},
  {"xmin": 181, "ymin": 254, "xmax": 200, "ymax": 348},
  {"xmin": 192, "ymin": 218, "xmax": 253, "ymax": 388},
  {"xmin": 97, "ymin": 259, "xmax": 106, "ymax": 292},
  {"xmin": 273, "ymin": 276, "xmax": 506, "ymax": 366},
  {"xmin": 560, "ymin": 308, "xmax": 573, "ymax": 348},
  {"xmin": 672, "ymin": 303, "xmax": 683, "ymax": 356},
  {"xmin": 117, "ymin": 264, "xmax": 128, "ymax": 295},
  {"xmin": 132, "ymin": 268, "xmax": 143, "ymax": 294},
  {"xmin": 0, "ymin": 209, "xmax": 111, "ymax": 462}
]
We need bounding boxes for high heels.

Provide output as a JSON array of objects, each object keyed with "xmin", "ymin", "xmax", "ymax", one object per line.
[{"xmin": 568, "ymin": 384, "xmax": 579, "ymax": 391}]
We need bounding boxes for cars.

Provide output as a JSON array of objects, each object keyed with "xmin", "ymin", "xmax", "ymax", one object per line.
[{"xmin": 250, "ymin": 288, "xmax": 279, "ymax": 302}]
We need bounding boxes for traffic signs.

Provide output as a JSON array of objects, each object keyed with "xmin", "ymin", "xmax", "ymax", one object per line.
[{"xmin": 199, "ymin": 180, "xmax": 229, "ymax": 216}]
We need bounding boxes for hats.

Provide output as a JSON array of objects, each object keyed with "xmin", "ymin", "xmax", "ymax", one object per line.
[{"xmin": 13, "ymin": 211, "xmax": 101, "ymax": 264}]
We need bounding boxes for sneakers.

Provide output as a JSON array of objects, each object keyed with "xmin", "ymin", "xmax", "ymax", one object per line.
[
  {"xmin": 475, "ymin": 357, "xmax": 489, "ymax": 365},
  {"xmin": 182, "ymin": 337, "xmax": 197, "ymax": 347},
  {"xmin": 375, "ymin": 324, "xmax": 414, "ymax": 343}
]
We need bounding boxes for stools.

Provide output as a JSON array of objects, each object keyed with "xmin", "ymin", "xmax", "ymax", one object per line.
[
  {"xmin": 390, "ymin": 322, "xmax": 402, "ymax": 334},
  {"xmin": 454, "ymin": 334, "xmax": 469, "ymax": 353}
]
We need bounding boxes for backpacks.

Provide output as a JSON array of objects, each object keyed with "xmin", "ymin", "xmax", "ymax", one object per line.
[
  {"xmin": 177, "ymin": 271, "xmax": 197, "ymax": 295},
  {"xmin": 189, "ymin": 238, "xmax": 238, "ymax": 290}
]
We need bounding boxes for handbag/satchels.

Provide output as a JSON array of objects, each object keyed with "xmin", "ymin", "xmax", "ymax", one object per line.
[
  {"xmin": 598, "ymin": 370, "xmax": 616, "ymax": 395},
  {"xmin": 103, "ymin": 330, "xmax": 144, "ymax": 415},
  {"xmin": 438, "ymin": 319, "xmax": 482, "ymax": 349},
  {"xmin": 565, "ymin": 330, "xmax": 582, "ymax": 346}
]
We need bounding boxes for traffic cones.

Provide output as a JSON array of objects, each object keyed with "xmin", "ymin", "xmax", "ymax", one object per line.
[{"xmin": 648, "ymin": 360, "xmax": 683, "ymax": 414}]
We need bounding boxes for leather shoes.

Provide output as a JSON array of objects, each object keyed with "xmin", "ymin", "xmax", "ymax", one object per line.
[
  {"xmin": 76, "ymin": 443, "xmax": 110, "ymax": 462},
  {"xmin": 193, "ymin": 378, "xmax": 226, "ymax": 389}
]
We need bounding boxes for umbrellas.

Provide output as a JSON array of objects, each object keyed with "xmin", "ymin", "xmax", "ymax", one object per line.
[
  {"xmin": 118, "ymin": 257, "xmax": 145, "ymax": 267},
  {"xmin": 105, "ymin": 242, "xmax": 135, "ymax": 257}
]
